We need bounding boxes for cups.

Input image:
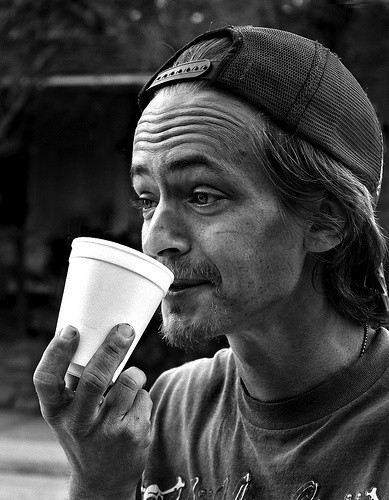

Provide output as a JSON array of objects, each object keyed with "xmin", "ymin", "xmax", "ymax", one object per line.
[{"xmin": 53, "ymin": 237, "xmax": 175, "ymax": 384}]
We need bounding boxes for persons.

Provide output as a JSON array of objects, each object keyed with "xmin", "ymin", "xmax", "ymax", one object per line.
[{"xmin": 33, "ymin": 24, "xmax": 389, "ymax": 500}]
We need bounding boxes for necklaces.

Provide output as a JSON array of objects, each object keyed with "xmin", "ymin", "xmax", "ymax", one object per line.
[{"xmin": 359, "ymin": 323, "xmax": 369, "ymax": 357}]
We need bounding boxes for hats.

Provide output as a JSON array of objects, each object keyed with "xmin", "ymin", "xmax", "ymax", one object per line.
[{"xmin": 137, "ymin": 24, "xmax": 389, "ymax": 313}]
